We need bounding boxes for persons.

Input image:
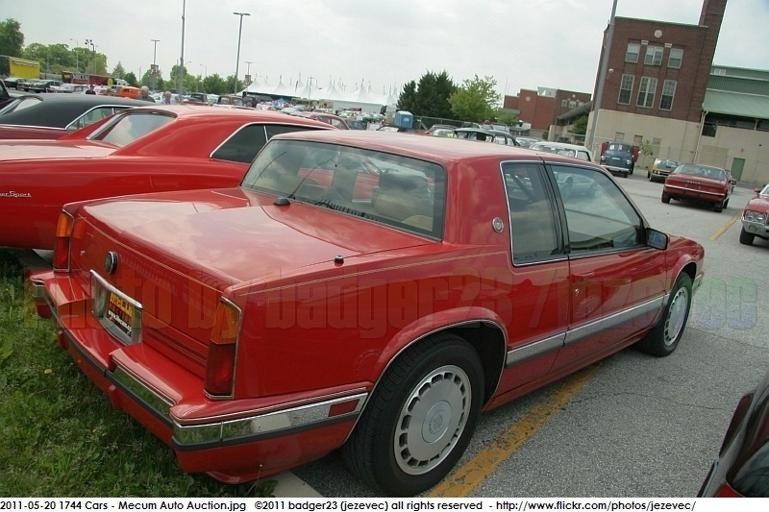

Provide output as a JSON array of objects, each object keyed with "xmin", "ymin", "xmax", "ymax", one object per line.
[
  {"xmin": 161, "ymin": 91, "xmax": 178, "ymax": 105},
  {"xmin": 86, "ymin": 84, "xmax": 96, "ymax": 95},
  {"xmin": 475, "ymin": 119, "xmax": 491, "ymax": 141},
  {"xmin": 135, "ymin": 85, "xmax": 157, "ymax": 102}
]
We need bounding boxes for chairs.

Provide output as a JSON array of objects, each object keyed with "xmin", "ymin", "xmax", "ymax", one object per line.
[{"xmin": 373, "ymin": 168, "xmax": 554, "ymax": 261}]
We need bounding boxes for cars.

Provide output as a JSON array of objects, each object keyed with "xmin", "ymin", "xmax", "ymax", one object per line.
[
  {"xmin": 0, "ymin": 103, "xmax": 386, "ymax": 255},
  {"xmin": 738, "ymin": 182, "xmax": 768, "ymax": 246},
  {"xmin": 661, "ymin": 161, "xmax": 733, "ymax": 212},
  {"xmin": 599, "ymin": 141, "xmax": 641, "ymax": 179},
  {"xmin": 647, "ymin": 157, "xmax": 679, "ymax": 183},
  {"xmin": 23, "ymin": 124, "xmax": 707, "ymax": 494}
]
